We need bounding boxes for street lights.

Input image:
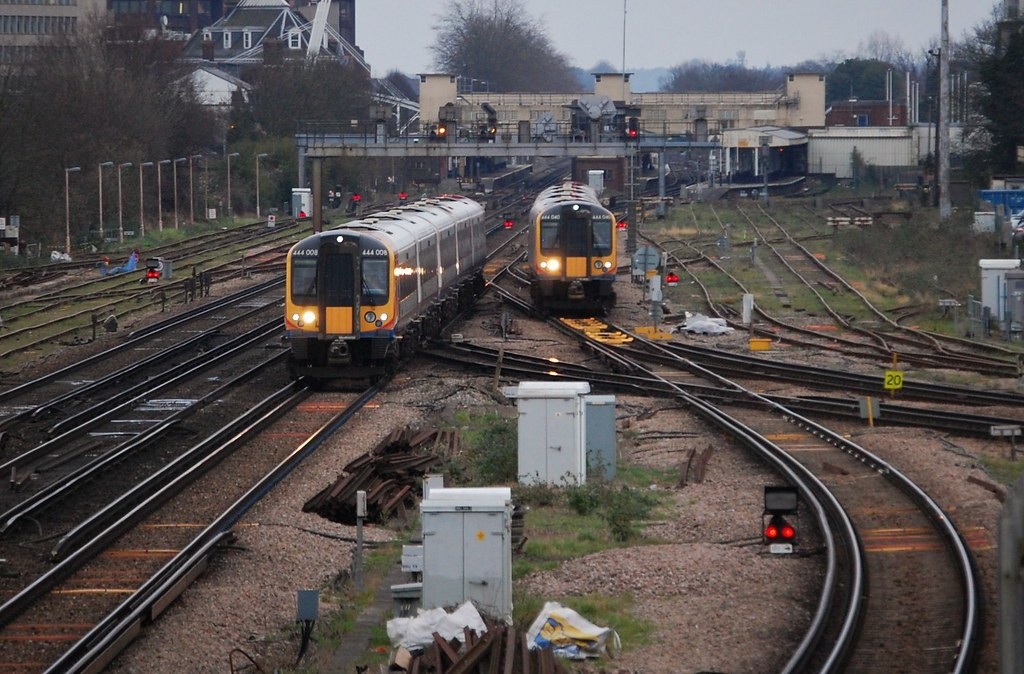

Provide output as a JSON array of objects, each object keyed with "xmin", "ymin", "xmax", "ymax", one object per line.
[{"xmin": 63, "ymin": 149, "xmax": 271, "ymax": 256}]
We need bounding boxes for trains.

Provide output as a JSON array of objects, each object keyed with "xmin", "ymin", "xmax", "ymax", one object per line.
[
  {"xmin": 527, "ymin": 180, "xmax": 618, "ymax": 321},
  {"xmin": 282, "ymin": 192, "xmax": 486, "ymax": 390}
]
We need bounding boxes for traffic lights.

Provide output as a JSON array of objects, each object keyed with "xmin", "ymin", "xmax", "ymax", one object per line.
[
  {"xmin": 488, "ymin": 116, "xmax": 496, "ymax": 139},
  {"xmin": 629, "ymin": 118, "xmax": 640, "ymax": 143},
  {"xmin": 439, "ymin": 120, "xmax": 446, "ymax": 141}
]
